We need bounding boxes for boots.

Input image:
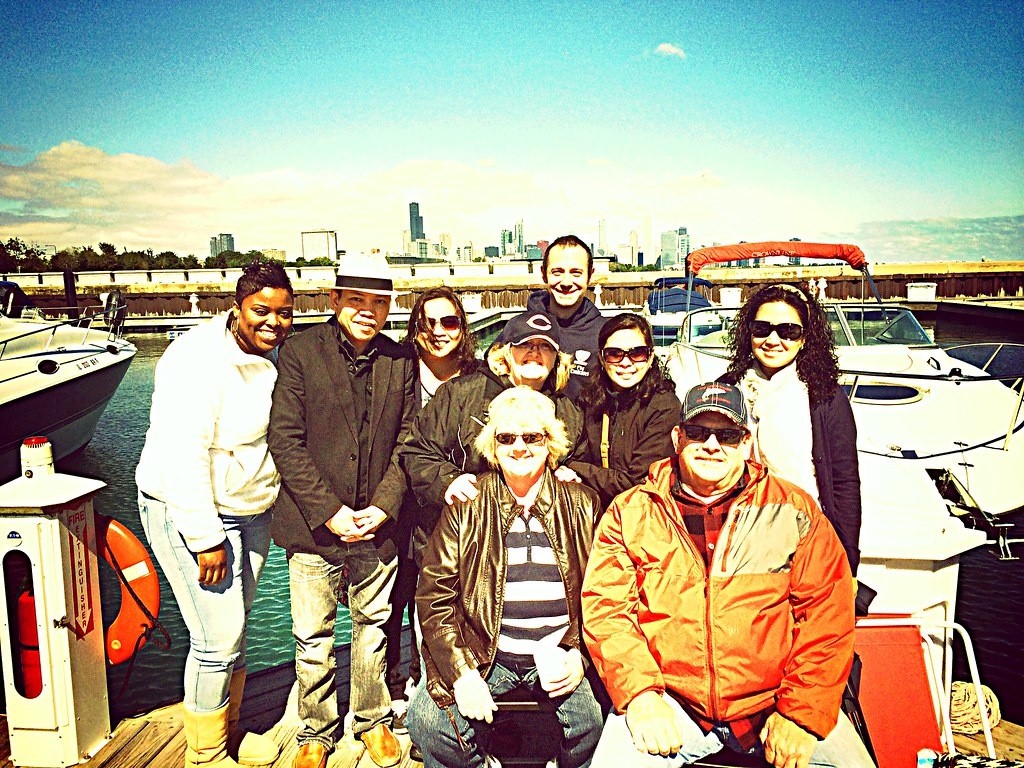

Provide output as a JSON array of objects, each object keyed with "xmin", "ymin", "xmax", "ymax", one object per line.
[
  {"xmin": 182, "ymin": 690, "xmax": 252, "ymax": 768},
  {"xmin": 226, "ymin": 664, "xmax": 279, "ymax": 766}
]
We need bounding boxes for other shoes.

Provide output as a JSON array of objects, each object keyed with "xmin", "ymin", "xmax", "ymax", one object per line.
[
  {"xmin": 410, "ymin": 742, "xmax": 423, "ymax": 762},
  {"xmin": 391, "ymin": 700, "xmax": 412, "ymax": 734}
]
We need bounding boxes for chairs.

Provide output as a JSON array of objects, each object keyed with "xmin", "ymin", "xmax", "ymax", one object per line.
[{"xmin": 846, "ymin": 601, "xmax": 994, "ymax": 768}]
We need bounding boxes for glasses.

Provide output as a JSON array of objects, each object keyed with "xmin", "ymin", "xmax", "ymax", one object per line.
[
  {"xmin": 513, "ymin": 342, "xmax": 555, "ymax": 351},
  {"xmin": 679, "ymin": 421, "xmax": 747, "ymax": 444},
  {"xmin": 602, "ymin": 346, "xmax": 654, "ymax": 363},
  {"xmin": 492, "ymin": 430, "xmax": 550, "ymax": 445},
  {"xmin": 749, "ymin": 321, "xmax": 804, "ymax": 341},
  {"xmin": 414, "ymin": 316, "xmax": 462, "ymax": 332}
]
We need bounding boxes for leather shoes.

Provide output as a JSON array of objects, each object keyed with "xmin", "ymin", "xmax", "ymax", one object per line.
[
  {"xmin": 359, "ymin": 722, "xmax": 402, "ymax": 768},
  {"xmin": 291, "ymin": 741, "xmax": 335, "ymax": 768}
]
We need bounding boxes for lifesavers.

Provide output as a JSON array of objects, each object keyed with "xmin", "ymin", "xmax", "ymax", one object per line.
[{"xmin": 96, "ymin": 514, "xmax": 161, "ymax": 668}]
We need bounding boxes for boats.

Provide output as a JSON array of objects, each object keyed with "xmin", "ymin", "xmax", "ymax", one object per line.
[
  {"xmin": 0, "ymin": 304, "xmax": 138, "ymax": 486},
  {"xmin": 643, "ymin": 277, "xmax": 727, "ymax": 341},
  {"xmin": 653, "ymin": 240, "xmax": 1023, "ymax": 519}
]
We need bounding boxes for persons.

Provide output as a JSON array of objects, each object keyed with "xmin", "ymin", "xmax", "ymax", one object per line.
[
  {"xmin": 550, "ymin": 310, "xmax": 683, "ymax": 518},
  {"xmin": 401, "ymin": 384, "xmax": 601, "ymax": 768},
  {"xmin": 526, "ymin": 233, "xmax": 605, "ymax": 408},
  {"xmin": 383, "ymin": 284, "xmax": 476, "ymax": 732},
  {"xmin": 716, "ymin": 279, "xmax": 862, "ymax": 598},
  {"xmin": 139, "ymin": 259, "xmax": 294, "ymax": 768},
  {"xmin": 267, "ymin": 251, "xmax": 416, "ymax": 768},
  {"xmin": 580, "ymin": 382, "xmax": 880, "ymax": 768},
  {"xmin": 401, "ymin": 308, "xmax": 590, "ymax": 760}
]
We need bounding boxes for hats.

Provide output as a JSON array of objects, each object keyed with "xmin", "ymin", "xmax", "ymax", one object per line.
[
  {"xmin": 679, "ymin": 382, "xmax": 748, "ymax": 427},
  {"xmin": 503, "ymin": 310, "xmax": 560, "ymax": 353},
  {"xmin": 317, "ymin": 252, "xmax": 411, "ymax": 295}
]
